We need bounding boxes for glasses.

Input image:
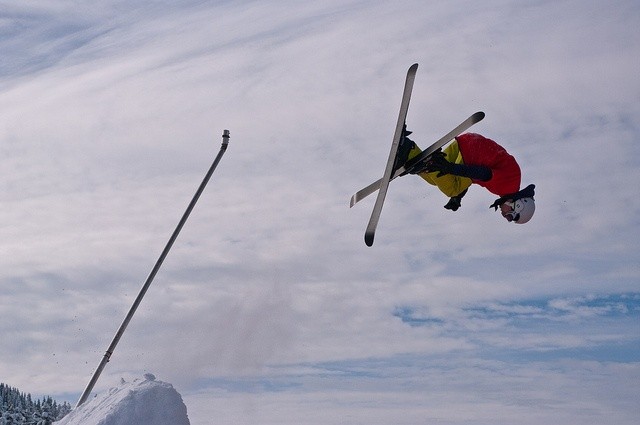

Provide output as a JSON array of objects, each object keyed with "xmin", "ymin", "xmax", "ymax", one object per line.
[{"xmin": 503, "ymin": 211, "xmax": 517, "ymax": 222}]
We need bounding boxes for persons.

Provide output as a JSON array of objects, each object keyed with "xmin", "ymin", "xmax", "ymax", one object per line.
[{"xmin": 391, "ymin": 132, "xmax": 536, "ymax": 224}]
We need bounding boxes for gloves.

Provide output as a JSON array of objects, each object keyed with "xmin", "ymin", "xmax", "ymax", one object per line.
[
  {"xmin": 444, "ymin": 188, "xmax": 467, "ymax": 212},
  {"xmin": 428, "ymin": 159, "xmax": 461, "ymax": 177}
]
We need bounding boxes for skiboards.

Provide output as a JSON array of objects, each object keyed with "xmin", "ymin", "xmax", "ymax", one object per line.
[{"xmin": 350, "ymin": 63, "xmax": 485, "ymax": 247}]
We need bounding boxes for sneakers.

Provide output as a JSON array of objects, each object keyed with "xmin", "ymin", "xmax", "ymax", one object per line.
[
  {"xmin": 396, "ymin": 136, "xmax": 413, "ymax": 171},
  {"xmin": 410, "ymin": 154, "xmax": 444, "ymax": 173}
]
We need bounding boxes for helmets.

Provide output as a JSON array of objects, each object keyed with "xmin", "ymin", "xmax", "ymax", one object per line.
[{"xmin": 503, "ymin": 198, "xmax": 535, "ymax": 224}]
